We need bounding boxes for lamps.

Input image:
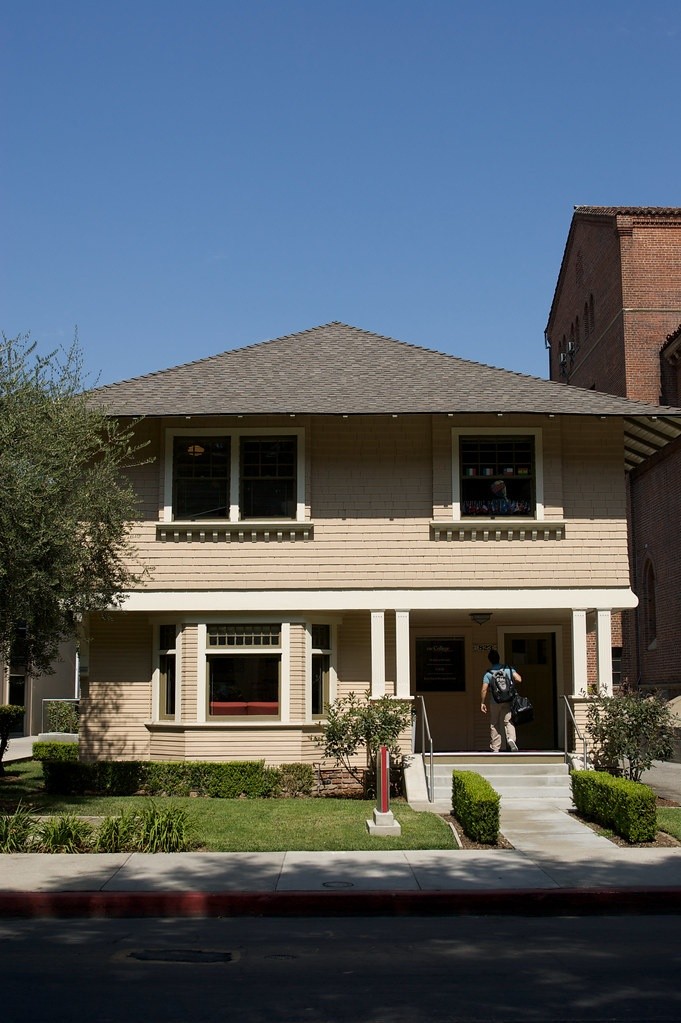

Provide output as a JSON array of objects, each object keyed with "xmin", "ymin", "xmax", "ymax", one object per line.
[{"xmin": 468, "ymin": 613, "xmax": 493, "ymax": 626}]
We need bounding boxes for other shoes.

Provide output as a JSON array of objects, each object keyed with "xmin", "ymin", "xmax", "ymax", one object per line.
[
  {"xmin": 493, "ymin": 748, "xmax": 500, "ymax": 753},
  {"xmin": 508, "ymin": 739, "xmax": 518, "ymax": 752}
]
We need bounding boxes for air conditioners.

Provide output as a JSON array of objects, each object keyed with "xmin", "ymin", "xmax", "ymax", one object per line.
[
  {"xmin": 559, "ymin": 353, "xmax": 566, "ymax": 365},
  {"xmin": 567, "ymin": 341, "xmax": 575, "ymax": 354}
]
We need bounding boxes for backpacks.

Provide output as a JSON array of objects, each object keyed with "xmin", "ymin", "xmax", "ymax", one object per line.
[{"xmin": 487, "ymin": 665, "xmax": 516, "ymax": 704}]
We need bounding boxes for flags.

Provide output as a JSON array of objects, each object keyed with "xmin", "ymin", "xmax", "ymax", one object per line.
[
  {"xmin": 491, "ymin": 479, "xmax": 512, "ymax": 498},
  {"xmin": 483, "ymin": 468, "xmax": 493, "ymax": 476},
  {"xmin": 465, "ymin": 468, "xmax": 476, "ymax": 476},
  {"xmin": 518, "ymin": 468, "xmax": 528, "ymax": 476},
  {"xmin": 503, "ymin": 467, "xmax": 513, "ymax": 476}
]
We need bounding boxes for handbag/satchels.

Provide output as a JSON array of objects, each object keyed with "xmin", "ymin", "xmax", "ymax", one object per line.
[{"xmin": 510, "ymin": 689, "xmax": 532, "ymax": 715}]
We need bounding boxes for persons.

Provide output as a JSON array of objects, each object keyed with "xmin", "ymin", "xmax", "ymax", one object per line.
[
  {"xmin": 480, "ymin": 649, "xmax": 521, "ymax": 752},
  {"xmin": 462, "ymin": 500, "xmax": 528, "ymax": 515}
]
